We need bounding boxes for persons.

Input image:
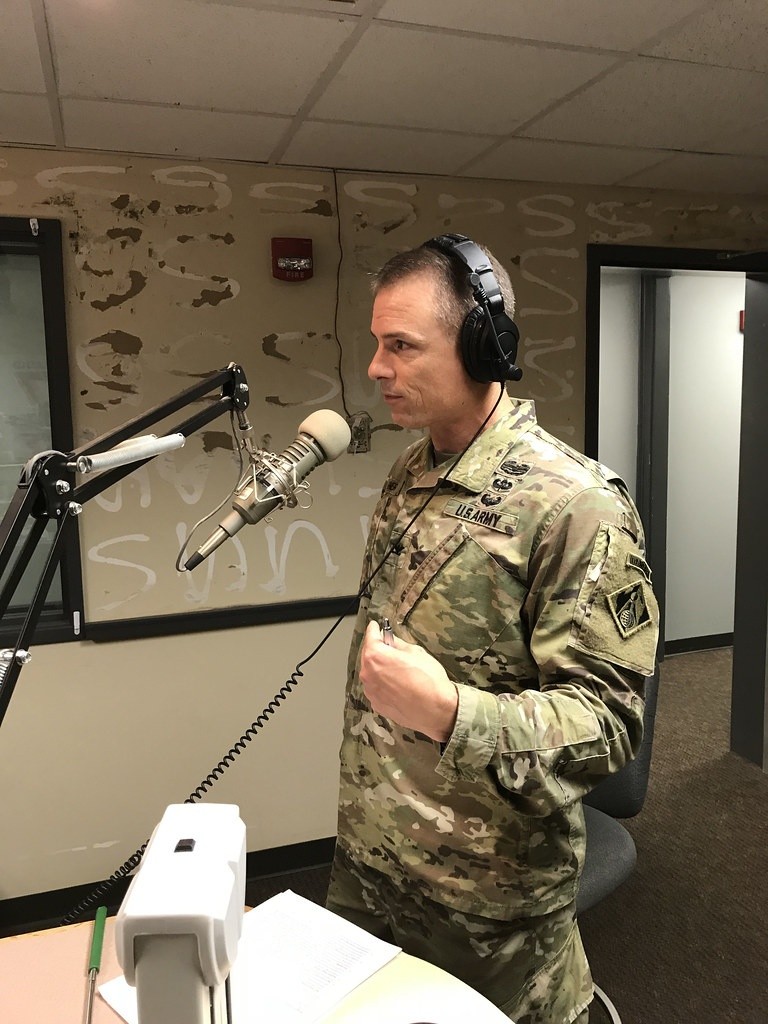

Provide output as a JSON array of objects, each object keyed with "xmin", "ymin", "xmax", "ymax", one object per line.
[{"xmin": 325, "ymin": 232, "xmax": 662, "ymax": 1024}]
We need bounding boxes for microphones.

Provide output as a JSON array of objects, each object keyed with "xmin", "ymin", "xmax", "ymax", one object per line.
[{"xmin": 183, "ymin": 409, "xmax": 352, "ymax": 571}]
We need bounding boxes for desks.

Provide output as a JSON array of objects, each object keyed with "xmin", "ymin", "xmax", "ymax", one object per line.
[{"xmin": 0, "ymin": 898, "xmax": 255, "ymax": 1024}]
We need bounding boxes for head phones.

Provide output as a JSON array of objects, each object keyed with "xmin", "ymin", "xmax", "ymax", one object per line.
[{"xmin": 423, "ymin": 233, "xmax": 523, "ymax": 385}]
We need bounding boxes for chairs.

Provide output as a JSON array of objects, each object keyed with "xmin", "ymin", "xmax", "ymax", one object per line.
[{"xmin": 574, "ymin": 659, "xmax": 659, "ymax": 1024}]
